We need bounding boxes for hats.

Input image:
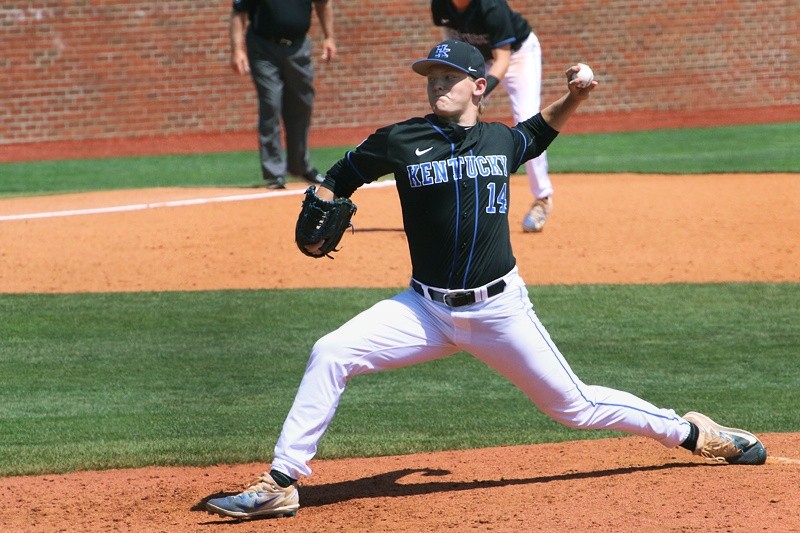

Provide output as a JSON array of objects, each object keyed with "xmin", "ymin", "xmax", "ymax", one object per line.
[{"xmin": 411, "ymin": 39, "xmax": 486, "ymax": 80}]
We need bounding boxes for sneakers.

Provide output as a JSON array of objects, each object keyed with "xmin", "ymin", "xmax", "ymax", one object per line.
[
  {"xmin": 681, "ymin": 410, "xmax": 767, "ymax": 465},
  {"xmin": 289, "ymin": 167, "xmax": 326, "ymax": 184},
  {"xmin": 522, "ymin": 196, "xmax": 553, "ymax": 232},
  {"xmin": 267, "ymin": 176, "xmax": 286, "ymax": 189},
  {"xmin": 205, "ymin": 472, "xmax": 300, "ymax": 520}
]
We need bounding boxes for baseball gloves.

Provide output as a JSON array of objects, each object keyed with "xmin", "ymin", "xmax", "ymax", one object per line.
[{"xmin": 295, "ymin": 184, "xmax": 357, "ymax": 259}]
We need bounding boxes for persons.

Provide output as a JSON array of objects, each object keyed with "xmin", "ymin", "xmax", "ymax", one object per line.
[
  {"xmin": 431, "ymin": 0, "xmax": 552, "ymax": 232},
  {"xmin": 207, "ymin": 38, "xmax": 767, "ymax": 521},
  {"xmin": 229, "ymin": 0, "xmax": 336, "ymax": 189}
]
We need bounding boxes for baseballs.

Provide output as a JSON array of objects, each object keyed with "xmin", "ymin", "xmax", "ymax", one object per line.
[{"xmin": 570, "ymin": 63, "xmax": 594, "ymax": 89}]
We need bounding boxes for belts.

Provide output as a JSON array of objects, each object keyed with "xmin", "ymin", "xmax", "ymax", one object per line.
[
  {"xmin": 264, "ymin": 33, "xmax": 300, "ymax": 45},
  {"xmin": 410, "ymin": 276, "xmax": 504, "ymax": 307}
]
